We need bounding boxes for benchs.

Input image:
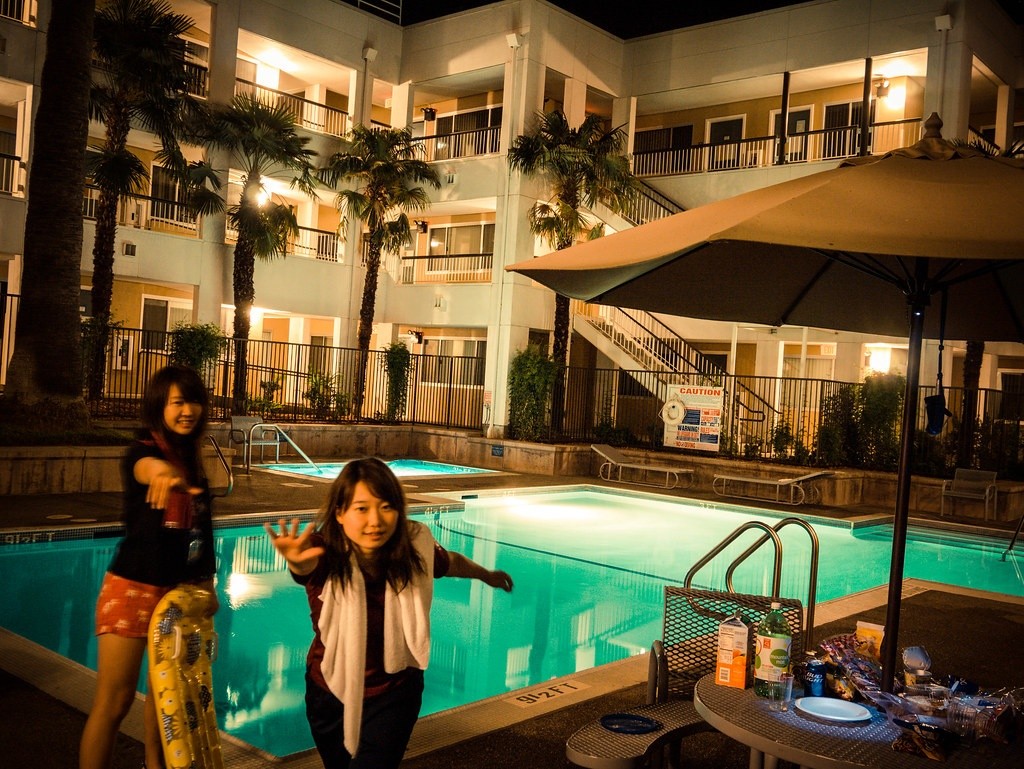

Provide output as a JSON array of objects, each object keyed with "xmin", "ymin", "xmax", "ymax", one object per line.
[{"xmin": 567, "ymin": 696, "xmax": 718, "ymax": 769}]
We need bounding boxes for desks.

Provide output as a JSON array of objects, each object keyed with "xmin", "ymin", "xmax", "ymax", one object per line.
[{"xmin": 693, "ymin": 672, "xmax": 1024, "ymax": 769}]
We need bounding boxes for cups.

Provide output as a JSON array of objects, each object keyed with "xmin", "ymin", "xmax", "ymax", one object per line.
[
  {"xmin": 162, "ymin": 489, "xmax": 192, "ymax": 528},
  {"xmin": 767, "ymin": 668, "xmax": 794, "ymax": 712},
  {"xmin": 904, "ymin": 669, "xmax": 932, "ymax": 697},
  {"xmin": 948, "ymin": 699, "xmax": 978, "ymax": 748}
]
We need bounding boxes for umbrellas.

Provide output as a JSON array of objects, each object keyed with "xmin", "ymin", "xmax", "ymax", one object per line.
[{"xmin": 503, "ymin": 113, "xmax": 1023, "ymax": 701}]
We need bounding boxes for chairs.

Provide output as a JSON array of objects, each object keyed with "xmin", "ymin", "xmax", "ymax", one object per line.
[
  {"xmin": 712, "ymin": 471, "xmax": 836, "ymax": 506},
  {"xmin": 227, "ymin": 416, "xmax": 280, "ymax": 465},
  {"xmin": 591, "ymin": 444, "xmax": 694, "ymax": 490},
  {"xmin": 940, "ymin": 468, "xmax": 999, "ymax": 522}
]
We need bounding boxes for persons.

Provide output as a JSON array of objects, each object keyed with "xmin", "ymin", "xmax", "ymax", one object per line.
[
  {"xmin": 263, "ymin": 458, "xmax": 515, "ymax": 769},
  {"xmin": 77, "ymin": 363, "xmax": 219, "ymax": 769}
]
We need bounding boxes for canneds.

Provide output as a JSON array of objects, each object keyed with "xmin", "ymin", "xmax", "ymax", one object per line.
[{"xmin": 803, "ymin": 659, "xmax": 825, "ymax": 696}]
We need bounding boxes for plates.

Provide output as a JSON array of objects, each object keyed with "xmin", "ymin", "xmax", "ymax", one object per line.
[{"xmin": 795, "ymin": 696, "xmax": 872, "ymax": 722}]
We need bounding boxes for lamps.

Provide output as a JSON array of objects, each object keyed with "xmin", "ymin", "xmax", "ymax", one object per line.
[
  {"xmin": 420, "ymin": 107, "xmax": 436, "ymax": 122},
  {"xmin": 874, "ymin": 78, "xmax": 890, "ymax": 96},
  {"xmin": 414, "ymin": 220, "xmax": 426, "ymax": 233},
  {"xmin": 407, "ymin": 330, "xmax": 422, "ymax": 344}
]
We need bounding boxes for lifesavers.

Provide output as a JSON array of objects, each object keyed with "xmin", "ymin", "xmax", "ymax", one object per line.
[{"xmin": 661, "ymin": 400, "xmax": 685, "ymax": 427}]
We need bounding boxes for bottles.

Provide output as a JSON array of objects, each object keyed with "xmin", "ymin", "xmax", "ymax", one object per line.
[{"xmin": 754, "ymin": 602, "xmax": 792, "ymax": 699}]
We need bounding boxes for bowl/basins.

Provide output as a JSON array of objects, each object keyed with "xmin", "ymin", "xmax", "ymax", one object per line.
[{"xmin": 906, "ymin": 684, "xmax": 951, "ymax": 708}]
